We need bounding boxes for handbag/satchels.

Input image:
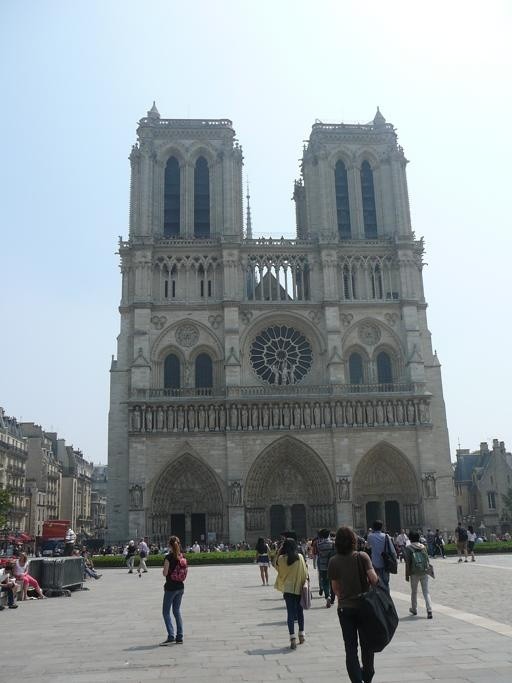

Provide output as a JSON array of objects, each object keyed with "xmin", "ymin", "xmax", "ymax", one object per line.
[
  {"xmin": 425, "ymin": 563, "xmax": 435, "ymax": 579},
  {"xmin": 357, "ymin": 578, "xmax": 399, "ymax": 653},
  {"xmin": 299, "ymin": 580, "xmax": 311, "ymax": 609},
  {"xmin": 140, "ymin": 551, "xmax": 146, "ymax": 558},
  {"xmin": 381, "ymin": 551, "xmax": 398, "ymax": 574}
]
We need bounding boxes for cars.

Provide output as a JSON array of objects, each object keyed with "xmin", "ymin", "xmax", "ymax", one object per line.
[{"xmin": 36, "ymin": 537, "xmax": 66, "ymax": 557}]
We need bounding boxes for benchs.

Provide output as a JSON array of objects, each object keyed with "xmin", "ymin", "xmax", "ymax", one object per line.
[
  {"xmin": 83, "ymin": 568, "xmax": 93, "ymax": 579},
  {"xmin": 0, "ymin": 579, "xmax": 24, "ymax": 609}
]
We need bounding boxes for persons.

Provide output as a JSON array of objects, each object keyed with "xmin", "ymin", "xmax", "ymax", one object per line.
[
  {"xmin": 185, "ymin": 541, "xmax": 251, "ymax": 553},
  {"xmin": 255, "ymin": 538, "xmax": 271, "ymax": 585},
  {"xmin": 74, "ymin": 549, "xmax": 103, "ymax": 580},
  {"xmin": 454, "ymin": 522, "xmax": 476, "ymax": 562},
  {"xmin": 365, "ymin": 520, "xmax": 447, "ymax": 618},
  {"xmin": 0, "ymin": 552, "xmax": 45, "ymax": 608},
  {"xmin": 298, "ymin": 527, "xmax": 336, "ymax": 608},
  {"xmin": 274, "ymin": 537, "xmax": 307, "ymax": 648},
  {"xmin": 159, "ymin": 536, "xmax": 184, "ymax": 646},
  {"xmin": 327, "ymin": 527, "xmax": 377, "ymax": 682},
  {"xmin": 100, "ymin": 544, "xmax": 128, "ymax": 555},
  {"xmin": 150, "ymin": 544, "xmax": 170, "ymax": 554},
  {"xmin": 126, "ymin": 539, "xmax": 149, "ymax": 574},
  {"xmin": 476, "ymin": 531, "xmax": 512, "ymax": 542}
]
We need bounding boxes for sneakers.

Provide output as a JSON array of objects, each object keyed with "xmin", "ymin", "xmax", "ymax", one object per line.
[
  {"xmin": 326, "ymin": 597, "xmax": 331, "ymax": 608},
  {"xmin": 319, "ymin": 587, "xmax": 323, "ymax": 596},
  {"xmin": 409, "ymin": 608, "xmax": 417, "ymax": 615},
  {"xmin": 143, "ymin": 571, "xmax": 147, "ymax": 573},
  {"xmin": 160, "ymin": 639, "xmax": 177, "ymax": 646},
  {"xmin": 9, "ymin": 605, "xmax": 18, "ymax": 608},
  {"xmin": 299, "ymin": 635, "xmax": 305, "ymax": 644},
  {"xmin": 176, "ymin": 639, "xmax": 183, "ymax": 643},
  {"xmin": 128, "ymin": 570, "xmax": 133, "ymax": 573},
  {"xmin": 95, "ymin": 575, "xmax": 102, "ymax": 579},
  {"xmin": 291, "ymin": 638, "xmax": 296, "ymax": 649},
  {"xmin": 38, "ymin": 595, "xmax": 47, "ymax": 599},
  {"xmin": 427, "ymin": 609, "xmax": 433, "ymax": 618}
]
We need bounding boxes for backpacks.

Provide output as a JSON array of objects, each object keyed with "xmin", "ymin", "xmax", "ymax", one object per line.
[
  {"xmin": 171, "ymin": 553, "xmax": 189, "ymax": 582},
  {"xmin": 411, "ymin": 551, "xmax": 429, "ymax": 575},
  {"xmin": 459, "ymin": 529, "xmax": 468, "ymax": 542}
]
